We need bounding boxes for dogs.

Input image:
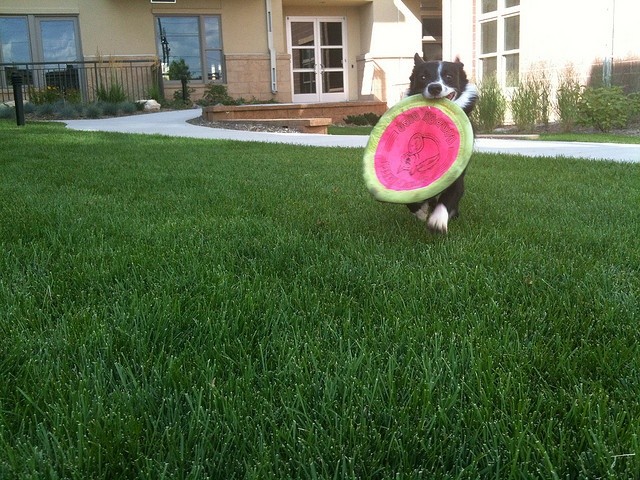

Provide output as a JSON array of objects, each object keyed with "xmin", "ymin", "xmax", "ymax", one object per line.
[{"xmin": 402, "ymin": 52, "xmax": 481, "ymax": 235}]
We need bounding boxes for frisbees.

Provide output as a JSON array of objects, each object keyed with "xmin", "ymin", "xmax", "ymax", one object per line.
[{"xmin": 360, "ymin": 93, "xmax": 474, "ymax": 203}]
